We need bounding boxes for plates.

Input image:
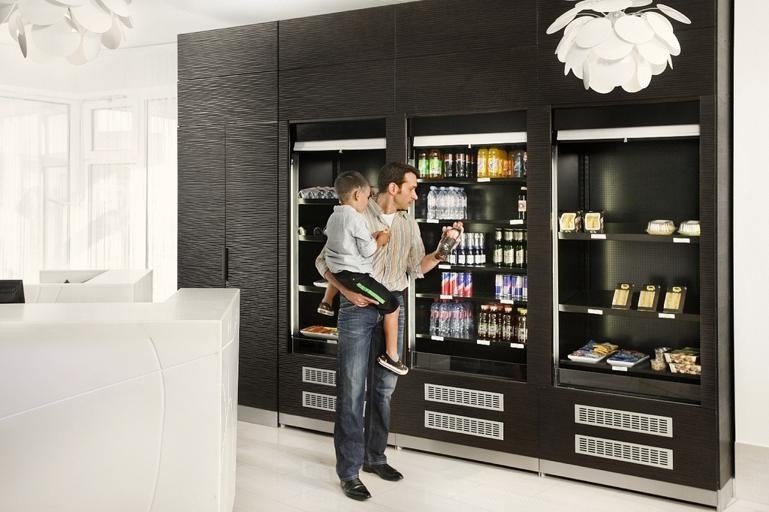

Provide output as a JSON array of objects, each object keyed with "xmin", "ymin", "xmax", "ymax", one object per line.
[
  {"xmin": 300, "ymin": 325, "xmax": 338, "ymax": 340},
  {"xmin": 567, "ymin": 344, "xmax": 651, "ymax": 368}
]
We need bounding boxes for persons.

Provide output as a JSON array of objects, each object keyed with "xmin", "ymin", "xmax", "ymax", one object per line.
[
  {"xmin": 317, "ymin": 169, "xmax": 409, "ymax": 375},
  {"xmin": 314, "ymin": 160, "xmax": 465, "ymax": 501}
]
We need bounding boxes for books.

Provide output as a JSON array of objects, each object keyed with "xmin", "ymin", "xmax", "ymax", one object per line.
[{"xmin": 567, "ymin": 339, "xmax": 650, "ymax": 367}]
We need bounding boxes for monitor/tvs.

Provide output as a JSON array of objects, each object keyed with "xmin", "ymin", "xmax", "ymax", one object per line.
[{"xmin": 0, "ymin": 280, "xmax": 25, "ymax": 304}]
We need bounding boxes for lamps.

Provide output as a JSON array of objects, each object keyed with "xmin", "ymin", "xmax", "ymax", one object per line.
[
  {"xmin": 549, "ymin": 0, "xmax": 691, "ymax": 93},
  {"xmin": 0, "ymin": 1, "xmax": 134, "ymax": 68}
]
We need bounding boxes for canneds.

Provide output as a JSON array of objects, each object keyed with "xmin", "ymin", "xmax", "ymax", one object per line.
[
  {"xmin": 441, "ymin": 271, "xmax": 473, "ymax": 298},
  {"xmin": 494, "ymin": 275, "xmax": 528, "ymax": 303}
]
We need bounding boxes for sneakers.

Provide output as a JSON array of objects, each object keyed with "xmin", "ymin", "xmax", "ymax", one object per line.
[
  {"xmin": 376, "ymin": 352, "xmax": 409, "ymax": 376},
  {"xmin": 317, "ymin": 302, "xmax": 334, "ymax": 316}
]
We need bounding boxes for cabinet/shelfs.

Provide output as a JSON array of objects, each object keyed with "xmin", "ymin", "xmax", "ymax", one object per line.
[
  {"xmin": 278, "ymin": 6, "xmax": 397, "ymax": 452},
  {"xmin": 393, "ymin": 1, "xmax": 542, "ymax": 476},
  {"xmin": 538, "ymin": 0, "xmax": 735, "ymax": 507},
  {"xmin": 179, "ymin": 22, "xmax": 282, "ymax": 430}
]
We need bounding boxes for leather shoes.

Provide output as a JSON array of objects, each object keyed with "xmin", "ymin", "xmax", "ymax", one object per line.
[
  {"xmin": 363, "ymin": 462, "xmax": 403, "ymax": 481},
  {"xmin": 341, "ymin": 478, "xmax": 372, "ymax": 500}
]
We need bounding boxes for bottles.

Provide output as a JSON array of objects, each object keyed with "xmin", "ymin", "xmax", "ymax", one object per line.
[
  {"xmin": 298, "ymin": 185, "xmax": 338, "ymax": 237},
  {"xmin": 417, "ymin": 148, "xmax": 527, "ymax": 342}
]
